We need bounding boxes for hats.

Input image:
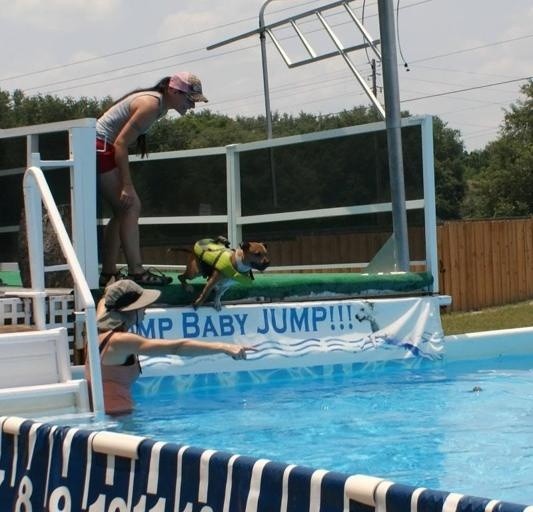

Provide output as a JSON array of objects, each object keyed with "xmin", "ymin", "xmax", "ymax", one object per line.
[
  {"xmin": 105, "ymin": 280, "xmax": 162, "ymax": 312},
  {"xmin": 170, "ymin": 73, "xmax": 208, "ymax": 103}
]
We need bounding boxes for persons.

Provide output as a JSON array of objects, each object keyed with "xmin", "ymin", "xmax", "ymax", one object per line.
[
  {"xmin": 81, "ymin": 275, "xmax": 262, "ymax": 418},
  {"xmin": 92, "ymin": 72, "xmax": 212, "ymax": 290}
]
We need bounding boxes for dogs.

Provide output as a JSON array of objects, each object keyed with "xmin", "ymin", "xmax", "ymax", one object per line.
[
  {"xmin": 166, "ymin": 235, "xmax": 271, "ymax": 313},
  {"xmin": 352, "ymin": 301, "xmax": 445, "ymax": 373}
]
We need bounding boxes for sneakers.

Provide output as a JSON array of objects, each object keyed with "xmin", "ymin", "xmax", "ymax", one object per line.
[
  {"xmin": 100, "ymin": 272, "xmax": 128, "ymax": 287},
  {"xmin": 128, "ymin": 269, "xmax": 173, "ymax": 285}
]
